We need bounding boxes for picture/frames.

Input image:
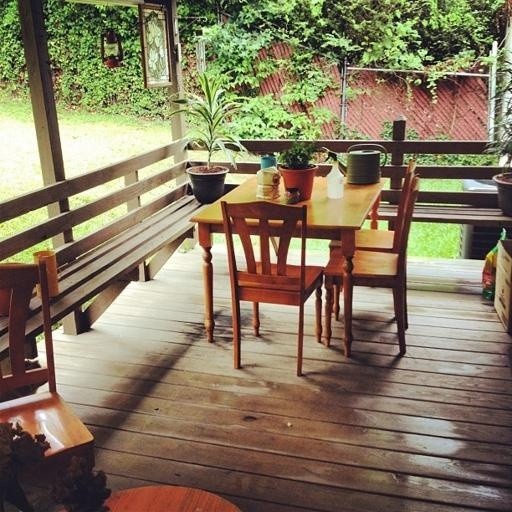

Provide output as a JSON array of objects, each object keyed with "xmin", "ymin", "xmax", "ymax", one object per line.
[{"xmin": 138, "ymin": 4, "xmax": 174, "ymax": 88}]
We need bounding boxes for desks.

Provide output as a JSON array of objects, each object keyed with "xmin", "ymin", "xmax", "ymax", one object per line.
[
  {"xmin": 190, "ymin": 170, "xmax": 385, "ymax": 358},
  {"xmin": 54, "ymin": 487, "xmax": 241, "ymax": 511}
]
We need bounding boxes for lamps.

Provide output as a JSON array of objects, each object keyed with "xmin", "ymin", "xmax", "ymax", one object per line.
[{"xmin": 99, "ymin": 25, "xmax": 126, "ymax": 68}]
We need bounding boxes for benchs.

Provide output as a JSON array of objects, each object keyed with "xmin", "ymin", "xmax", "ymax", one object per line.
[
  {"xmin": 366, "ymin": 207, "xmax": 510, "ymax": 231},
  {"xmin": 0, "ymin": 195, "xmax": 203, "ymax": 373}
]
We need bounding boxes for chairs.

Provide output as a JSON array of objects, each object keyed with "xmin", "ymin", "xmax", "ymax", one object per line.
[
  {"xmin": 329, "ymin": 159, "xmax": 416, "ymax": 313},
  {"xmin": 324, "ymin": 174, "xmax": 421, "ymax": 357},
  {"xmin": 0, "ymin": 261, "xmax": 96, "ymax": 468},
  {"xmin": 219, "ymin": 202, "xmax": 323, "ymax": 376}
]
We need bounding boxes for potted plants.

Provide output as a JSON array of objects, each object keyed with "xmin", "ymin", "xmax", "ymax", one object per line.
[
  {"xmin": 276, "ymin": 137, "xmax": 322, "ymax": 201},
  {"xmin": 482, "ymin": 48, "xmax": 512, "ymax": 214},
  {"xmin": 170, "ymin": 68, "xmax": 254, "ymax": 204}
]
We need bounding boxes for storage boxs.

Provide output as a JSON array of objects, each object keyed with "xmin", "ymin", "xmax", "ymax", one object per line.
[{"xmin": 494, "ymin": 239, "xmax": 511, "ymax": 334}]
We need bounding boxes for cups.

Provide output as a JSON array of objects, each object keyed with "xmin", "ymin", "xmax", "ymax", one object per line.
[
  {"xmin": 283, "ymin": 188, "xmax": 301, "ymax": 204},
  {"xmin": 32, "ymin": 250, "xmax": 59, "ymax": 299}
]
